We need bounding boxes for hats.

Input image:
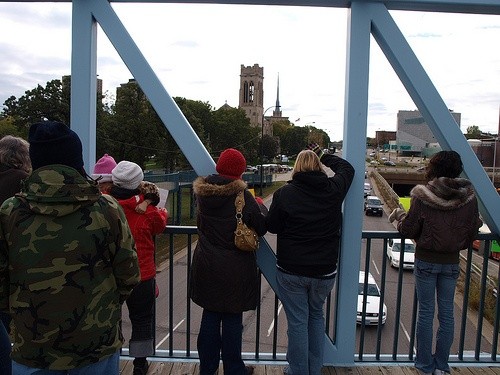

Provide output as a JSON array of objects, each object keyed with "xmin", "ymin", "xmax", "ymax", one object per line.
[
  {"xmin": 216, "ymin": 148, "xmax": 246, "ymax": 179},
  {"xmin": 430, "ymin": 150, "xmax": 463, "ymax": 177},
  {"xmin": 92, "ymin": 154, "xmax": 118, "ymax": 183},
  {"xmin": 27, "ymin": 112, "xmax": 88, "ymax": 177},
  {"xmin": 111, "ymin": 161, "xmax": 144, "ymax": 189}
]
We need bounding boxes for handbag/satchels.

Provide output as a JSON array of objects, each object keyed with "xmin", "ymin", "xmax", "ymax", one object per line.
[{"xmin": 234, "ymin": 190, "xmax": 260, "ymax": 250}]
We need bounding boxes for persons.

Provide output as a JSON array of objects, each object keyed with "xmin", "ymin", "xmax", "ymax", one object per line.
[
  {"xmin": 99, "ymin": 159, "xmax": 168, "ymax": 375},
  {"xmin": 388, "ymin": 151, "xmax": 483, "ymax": 375},
  {"xmin": 188, "ymin": 148, "xmax": 267, "ymax": 375},
  {"xmin": 0, "ymin": 120, "xmax": 141, "ymax": 375},
  {"xmin": 88, "ymin": 154, "xmax": 161, "ymax": 297},
  {"xmin": 267, "ymin": 142, "xmax": 355, "ymax": 375},
  {"xmin": 1, "ymin": 135, "xmax": 32, "ymax": 375}
]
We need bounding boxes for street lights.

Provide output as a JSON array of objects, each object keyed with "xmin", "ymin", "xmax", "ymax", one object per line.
[
  {"xmin": 259, "ymin": 105, "xmax": 282, "ymax": 196},
  {"xmin": 481, "ymin": 133, "xmax": 497, "ymax": 184}
]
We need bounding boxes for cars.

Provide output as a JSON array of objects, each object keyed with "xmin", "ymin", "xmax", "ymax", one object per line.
[
  {"xmin": 364, "ymin": 183, "xmax": 373, "ymax": 198},
  {"xmin": 370, "ymin": 148, "xmax": 396, "ymax": 167},
  {"xmin": 387, "ymin": 239, "xmax": 416, "ymax": 271},
  {"xmin": 245, "ymin": 154, "xmax": 295, "ymax": 173},
  {"xmin": 356, "ymin": 270, "xmax": 387, "ymax": 327},
  {"xmin": 417, "ymin": 166, "xmax": 428, "ymax": 172}
]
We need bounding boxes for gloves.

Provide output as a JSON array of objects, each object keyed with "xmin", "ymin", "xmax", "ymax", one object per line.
[
  {"xmin": 389, "ymin": 207, "xmax": 407, "ymax": 223},
  {"xmin": 305, "ymin": 141, "xmax": 326, "ymax": 160}
]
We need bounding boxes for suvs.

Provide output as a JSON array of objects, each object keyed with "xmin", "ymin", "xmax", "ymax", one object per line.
[{"xmin": 365, "ymin": 196, "xmax": 384, "ymax": 217}]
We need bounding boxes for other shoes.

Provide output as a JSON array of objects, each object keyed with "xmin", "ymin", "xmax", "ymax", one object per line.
[
  {"xmin": 283, "ymin": 365, "xmax": 293, "ymax": 374},
  {"xmin": 133, "ymin": 359, "xmax": 148, "ymax": 375},
  {"xmin": 416, "ymin": 368, "xmax": 432, "ymax": 375},
  {"xmin": 434, "ymin": 369, "xmax": 446, "ymax": 375},
  {"xmin": 246, "ymin": 365, "xmax": 254, "ymax": 375}
]
